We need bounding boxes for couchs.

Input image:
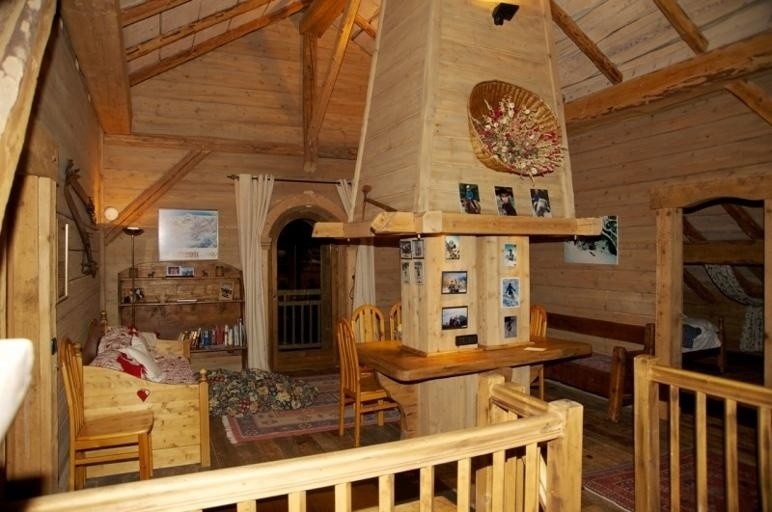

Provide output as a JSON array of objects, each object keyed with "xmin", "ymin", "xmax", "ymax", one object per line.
[{"xmin": 71, "ymin": 311, "xmax": 214, "ymax": 479}]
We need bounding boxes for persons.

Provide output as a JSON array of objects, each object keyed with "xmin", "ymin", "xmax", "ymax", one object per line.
[
  {"xmin": 505, "ymin": 283, "xmax": 516, "ymax": 299},
  {"xmin": 465, "ymin": 185, "xmax": 476, "ymax": 211},
  {"xmin": 506, "ymin": 248, "xmax": 514, "ymax": 261},
  {"xmin": 496, "ymin": 189, "xmax": 517, "ymax": 215},
  {"xmin": 533, "ymin": 190, "xmax": 550, "ymax": 212},
  {"xmin": 448, "ymin": 279, "xmax": 460, "ymax": 293}
]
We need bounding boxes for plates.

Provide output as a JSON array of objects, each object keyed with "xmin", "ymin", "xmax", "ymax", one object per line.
[{"xmin": 177, "ymin": 299, "xmax": 198, "ymax": 303}]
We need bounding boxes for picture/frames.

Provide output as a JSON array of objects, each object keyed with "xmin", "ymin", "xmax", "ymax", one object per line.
[
  {"xmin": 156, "ymin": 205, "xmax": 220, "ymax": 262},
  {"xmin": 399, "ymin": 237, "xmax": 424, "ymax": 284},
  {"xmin": 440, "ymin": 270, "xmax": 469, "ymax": 295},
  {"xmin": 441, "ymin": 306, "xmax": 469, "ymax": 330}
]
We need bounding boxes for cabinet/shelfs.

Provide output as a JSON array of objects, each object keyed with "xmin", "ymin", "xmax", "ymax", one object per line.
[{"xmin": 116, "ymin": 258, "xmax": 249, "ymax": 374}]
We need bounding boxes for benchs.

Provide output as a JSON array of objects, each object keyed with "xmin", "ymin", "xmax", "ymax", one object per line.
[{"xmin": 545, "ymin": 304, "xmax": 655, "ymax": 424}]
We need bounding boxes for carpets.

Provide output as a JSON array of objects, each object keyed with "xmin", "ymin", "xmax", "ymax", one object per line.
[
  {"xmin": 220, "ymin": 366, "xmax": 398, "ymax": 448},
  {"xmin": 585, "ymin": 440, "xmax": 766, "ymax": 512}
]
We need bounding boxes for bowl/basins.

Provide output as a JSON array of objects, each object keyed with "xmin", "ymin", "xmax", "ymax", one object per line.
[{"xmin": 156, "ymin": 295, "xmax": 170, "ymax": 303}]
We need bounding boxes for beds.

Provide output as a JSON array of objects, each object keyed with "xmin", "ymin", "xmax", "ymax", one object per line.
[{"xmin": 679, "ymin": 304, "xmax": 726, "ymax": 372}]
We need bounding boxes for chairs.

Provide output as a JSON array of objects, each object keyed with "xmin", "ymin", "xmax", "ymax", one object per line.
[
  {"xmin": 59, "ymin": 329, "xmax": 157, "ymax": 491},
  {"xmin": 334, "ymin": 315, "xmax": 400, "ymax": 444},
  {"xmin": 387, "ymin": 300, "xmax": 403, "ymax": 344},
  {"xmin": 524, "ymin": 303, "xmax": 549, "ymax": 402},
  {"xmin": 349, "ymin": 303, "xmax": 386, "ymax": 375}
]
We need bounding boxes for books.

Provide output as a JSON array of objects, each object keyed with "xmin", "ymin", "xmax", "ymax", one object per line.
[
  {"xmin": 178, "ymin": 319, "xmax": 246, "ymax": 348},
  {"xmin": 178, "ymin": 297, "xmax": 197, "ymax": 301}
]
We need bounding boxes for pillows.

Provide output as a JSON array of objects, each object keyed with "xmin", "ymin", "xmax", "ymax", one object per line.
[{"xmin": 115, "ymin": 333, "xmax": 163, "ymax": 383}]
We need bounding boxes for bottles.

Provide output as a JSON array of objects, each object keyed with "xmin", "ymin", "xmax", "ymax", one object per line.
[
  {"xmin": 130, "ymin": 268, "xmax": 138, "ymax": 278},
  {"xmin": 215, "ymin": 265, "xmax": 224, "ymax": 277}
]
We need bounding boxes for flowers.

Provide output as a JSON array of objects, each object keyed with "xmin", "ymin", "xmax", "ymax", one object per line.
[{"xmin": 477, "ymin": 94, "xmax": 563, "ymax": 178}]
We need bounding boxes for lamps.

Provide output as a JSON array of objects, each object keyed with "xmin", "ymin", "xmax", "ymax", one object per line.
[
  {"xmin": 124, "ymin": 222, "xmax": 145, "ymax": 332},
  {"xmin": 490, "ymin": 3, "xmax": 522, "ymax": 26}
]
